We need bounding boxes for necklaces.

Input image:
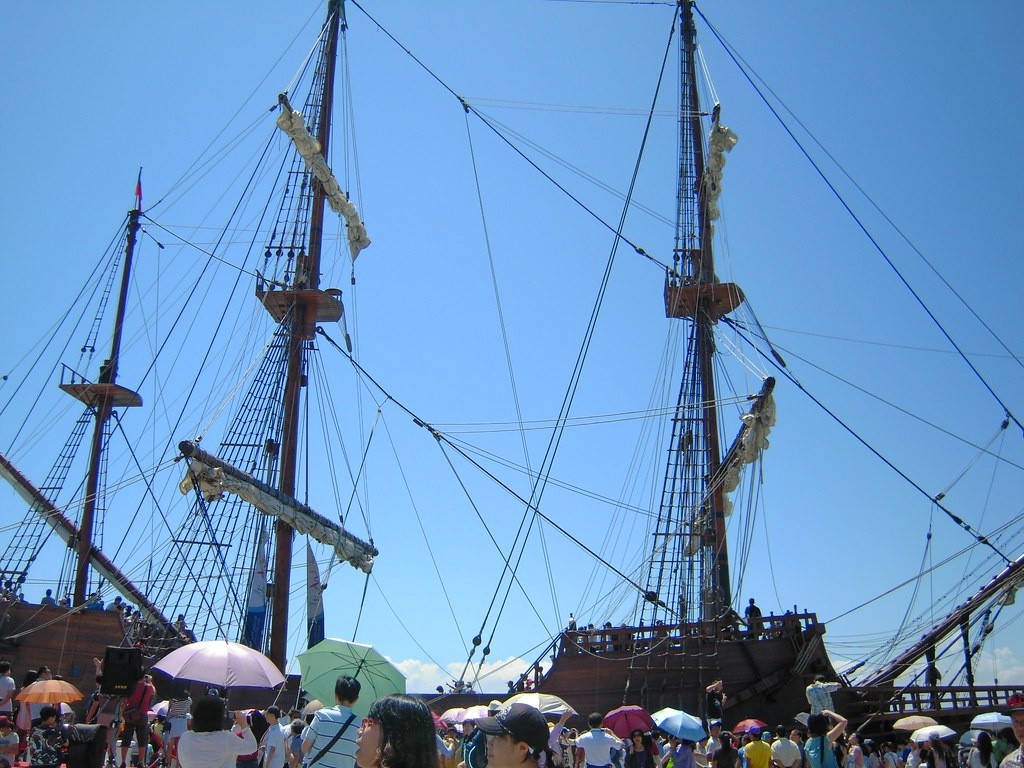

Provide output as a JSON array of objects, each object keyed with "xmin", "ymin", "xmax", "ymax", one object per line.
[{"xmin": 636, "ymin": 745, "xmax": 642, "ymax": 750}]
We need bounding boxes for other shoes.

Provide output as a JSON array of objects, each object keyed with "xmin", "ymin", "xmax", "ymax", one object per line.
[
  {"xmin": 137, "ymin": 763, "xmax": 144, "ymax": 768},
  {"xmin": 120, "ymin": 763, "xmax": 126, "ymax": 768}
]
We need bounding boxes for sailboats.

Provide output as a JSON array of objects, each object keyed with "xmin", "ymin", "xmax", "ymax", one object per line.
[
  {"xmin": 127, "ymin": 0, "xmax": 380, "ymax": 716},
  {"xmin": 0, "ymin": 166, "xmax": 197, "ymax": 723},
  {"xmin": 389, "ymin": 1, "xmax": 1024, "ymax": 735}
]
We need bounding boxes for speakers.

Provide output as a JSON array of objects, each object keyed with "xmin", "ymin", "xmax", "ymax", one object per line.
[{"xmin": 101, "ymin": 646, "xmax": 139, "ymax": 696}]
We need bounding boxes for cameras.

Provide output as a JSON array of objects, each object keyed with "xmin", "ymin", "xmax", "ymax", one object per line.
[
  {"xmin": 145, "ymin": 675, "xmax": 148, "ymax": 679},
  {"xmin": 227, "ymin": 711, "xmax": 236, "ymax": 719},
  {"xmin": 601, "ymin": 728, "xmax": 607, "ymax": 732}
]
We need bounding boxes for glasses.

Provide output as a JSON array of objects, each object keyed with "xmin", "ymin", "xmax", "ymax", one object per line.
[
  {"xmin": 633, "ymin": 734, "xmax": 642, "ymax": 737},
  {"xmin": 362, "ymin": 718, "xmax": 384, "ymax": 729}
]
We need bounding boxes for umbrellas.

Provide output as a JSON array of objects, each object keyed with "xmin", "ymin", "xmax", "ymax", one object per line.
[
  {"xmin": 892, "ymin": 716, "xmax": 938, "ymax": 730},
  {"xmin": 495, "ymin": 692, "xmax": 579, "ymax": 715},
  {"xmin": 602, "ymin": 705, "xmax": 655, "ymax": 740},
  {"xmin": 295, "ymin": 638, "xmax": 407, "ymax": 720},
  {"xmin": 969, "ymin": 712, "xmax": 1013, "ymax": 736},
  {"xmin": 150, "ymin": 641, "xmax": 288, "ymax": 691},
  {"xmin": 439, "ymin": 707, "xmax": 465, "ymax": 724},
  {"xmin": 457, "ymin": 705, "xmax": 489, "ymax": 723},
  {"xmin": 651, "ymin": 707, "xmax": 708, "ymax": 742},
  {"xmin": 732, "ymin": 716, "xmax": 769, "ymax": 734},
  {"xmin": 15, "ymin": 680, "xmax": 84, "ymax": 709},
  {"xmin": 151, "ymin": 700, "xmax": 192, "ymax": 720},
  {"xmin": 910, "ymin": 724, "xmax": 957, "ymax": 742}
]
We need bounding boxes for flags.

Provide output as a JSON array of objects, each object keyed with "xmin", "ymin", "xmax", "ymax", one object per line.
[{"xmin": 135, "ymin": 173, "xmax": 143, "ymax": 211}]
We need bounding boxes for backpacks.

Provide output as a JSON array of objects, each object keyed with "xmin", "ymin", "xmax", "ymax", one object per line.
[{"xmin": 463, "ymin": 727, "xmax": 488, "ymax": 768}]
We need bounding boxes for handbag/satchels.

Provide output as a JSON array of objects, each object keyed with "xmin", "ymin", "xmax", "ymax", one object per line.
[
  {"xmin": 122, "ymin": 708, "xmax": 140, "ymax": 724},
  {"xmin": 845, "ymin": 755, "xmax": 855, "ymax": 768}
]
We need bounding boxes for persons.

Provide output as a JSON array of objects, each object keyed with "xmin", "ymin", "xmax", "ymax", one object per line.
[
  {"xmin": 0, "ymin": 577, "xmax": 362, "ymax": 768},
  {"xmin": 355, "ymin": 693, "xmax": 442, "ymax": 768},
  {"xmin": 431, "ymin": 599, "xmax": 1024, "ymax": 768}
]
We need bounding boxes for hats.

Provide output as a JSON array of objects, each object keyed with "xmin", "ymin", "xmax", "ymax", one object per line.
[
  {"xmin": 1001, "ymin": 693, "xmax": 1024, "ymax": 716},
  {"xmin": 745, "ymin": 726, "xmax": 761, "ymax": 734},
  {"xmin": 266, "ymin": 706, "xmax": 279, "ymax": 714},
  {"xmin": 794, "ymin": 712, "xmax": 810, "ymax": 726},
  {"xmin": 303, "ymin": 699, "xmax": 324, "ymax": 714},
  {"xmin": 710, "ymin": 721, "xmax": 722, "ymax": 725},
  {"xmin": 488, "ymin": 700, "xmax": 502, "ymax": 710},
  {"xmin": 0, "ymin": 716, "xmax": 10, "ymax": 727},
  {"xmin": 928, "ymin": 731, "xmax": 940, "ymax": 740},
  {"xmin": 475, "ymin": 703, "xmax": 550, "ymax": 753},
  {"xmin": 864, "ymin": 739, "xmax": 874, "ymax": 744},
  {"xmin": 562, "ymin": 727, "xmax": 570, "ymax": 733}
]
